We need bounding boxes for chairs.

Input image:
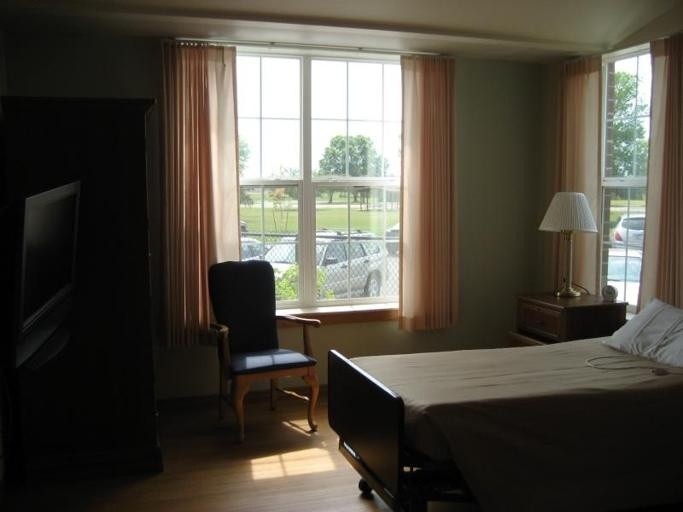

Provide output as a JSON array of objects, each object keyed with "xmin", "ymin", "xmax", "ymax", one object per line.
[{"xmin": 207, "ymin": 259, "xmax": 322, "ymax": 444}]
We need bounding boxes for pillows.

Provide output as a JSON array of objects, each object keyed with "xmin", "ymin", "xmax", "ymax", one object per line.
[{"xmin": 599, "ymin": 297, "xmax": 683, "ymax": 369}]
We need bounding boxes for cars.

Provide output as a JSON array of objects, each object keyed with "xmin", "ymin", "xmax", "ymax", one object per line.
[
  {"xmin": 385, "ymin": 223, "xmax": 400, "ymax": 254},
  {"xmin": 240, "ymin": 237, "xmax": 271, "ymax": 263},
  {"xmin": 607, "ymin": 248, "xmax": 642, "ymax": 306},
  {"xmin": 611, "ymin": 215, "xmax": 645, "ymax": 247}
]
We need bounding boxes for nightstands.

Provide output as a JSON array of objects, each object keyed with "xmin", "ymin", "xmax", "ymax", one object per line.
[{"xmin": 517, "ymin": 293, "xmax": 628, "ymax": 345}]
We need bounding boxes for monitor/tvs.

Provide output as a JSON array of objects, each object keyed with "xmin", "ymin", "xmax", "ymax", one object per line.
[{"xmin": 0, "ymin": 180, "xmax": 82, "ymax": 369}]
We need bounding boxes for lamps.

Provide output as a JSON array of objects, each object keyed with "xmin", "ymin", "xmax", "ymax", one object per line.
[{"xmin": 538, "ymin": 192, "xmax": 599, "ymax": 298}]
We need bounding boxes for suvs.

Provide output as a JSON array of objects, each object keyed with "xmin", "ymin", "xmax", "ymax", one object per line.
[{"xmin": 261, "ymin": 229, "xmax": 389, "ymax": 300}]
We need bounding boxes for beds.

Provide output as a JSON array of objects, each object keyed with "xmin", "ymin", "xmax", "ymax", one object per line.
[{"xmin": 328, "ymin": 336, "xmax": 683, "ymax": 512}]
